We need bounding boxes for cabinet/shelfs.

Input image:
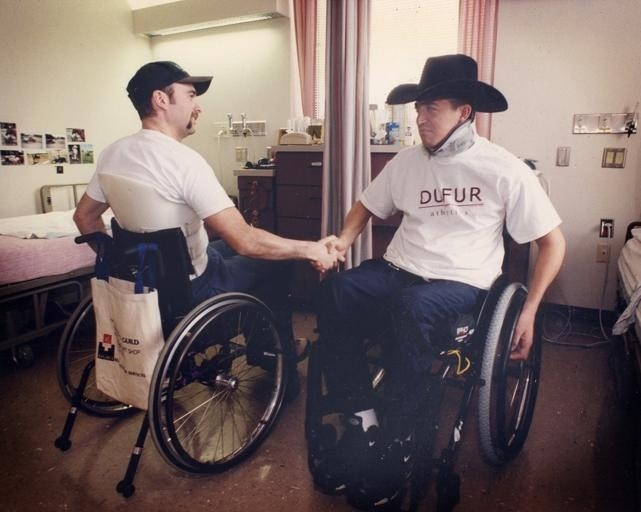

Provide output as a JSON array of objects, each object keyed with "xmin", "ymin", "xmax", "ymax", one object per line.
[
  {"xmin": 238, "ymin": 175, "xmax": 274, "ymax": 234},
  {"xmin": 275, "ymin": 153, "xmax": 323, "ymax": 242}
]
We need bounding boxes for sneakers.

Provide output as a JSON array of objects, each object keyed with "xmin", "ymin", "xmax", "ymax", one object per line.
[
  {"xmin": 247, "ymin": 335, "xmax": 310, "ymax": 370},
  {"xmin": 349, "ymin": 433, "xmax": 417, "ymax": 509},
  {"xmin": 312, "ymin": 413, "xmax": 379, "ymax": 495}
]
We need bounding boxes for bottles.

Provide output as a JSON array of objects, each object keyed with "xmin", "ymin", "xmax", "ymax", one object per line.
[
  {"xmin": 404, "ymin": 126, "xmax": 413, "ymax": 145},
  {"xmin": 388, "ymin": 122, "xmax": 400, "ymax": 144}
]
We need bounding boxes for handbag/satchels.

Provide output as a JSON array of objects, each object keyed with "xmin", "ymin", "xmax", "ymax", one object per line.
[{"xmin": 91, "ymin": 232, "xmax": 170, "ymax": 411}]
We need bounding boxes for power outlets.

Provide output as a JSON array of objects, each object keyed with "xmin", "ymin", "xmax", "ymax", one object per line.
[{"xmin": 600, "ymin": 219, "xmax": 614, "ymax": 237}]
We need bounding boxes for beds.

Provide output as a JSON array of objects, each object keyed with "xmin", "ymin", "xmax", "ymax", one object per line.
[{"xmin": 0, "ymin": 206, "xmax": 111, "ymax": 367}]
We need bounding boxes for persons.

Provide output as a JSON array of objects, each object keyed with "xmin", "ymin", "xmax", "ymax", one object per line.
[
  {"xmin": 69, "ymin": 61, "xmax": 348, "ymax": 373},
  {"xmin": 310, "ymin": 53, "xmax": 568, "ymax": 510}
]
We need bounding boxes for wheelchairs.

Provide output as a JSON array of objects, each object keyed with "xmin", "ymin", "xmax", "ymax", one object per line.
[
  {"xmin": 53, "ymin": 218, "xmax": 302, "ymax": 499},
  {"xmin": 305, "ymin": 222, "xmax": 542, "ymax": 511}
]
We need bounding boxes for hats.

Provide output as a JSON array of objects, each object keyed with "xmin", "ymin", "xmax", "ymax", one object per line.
[
  {"xmin": 387, "ymin": 54, "xmax": 508, "ymax": 112},
  {"xmin": 127, "ymin": 62, "xmax": 212, "ymax": 101}
]
